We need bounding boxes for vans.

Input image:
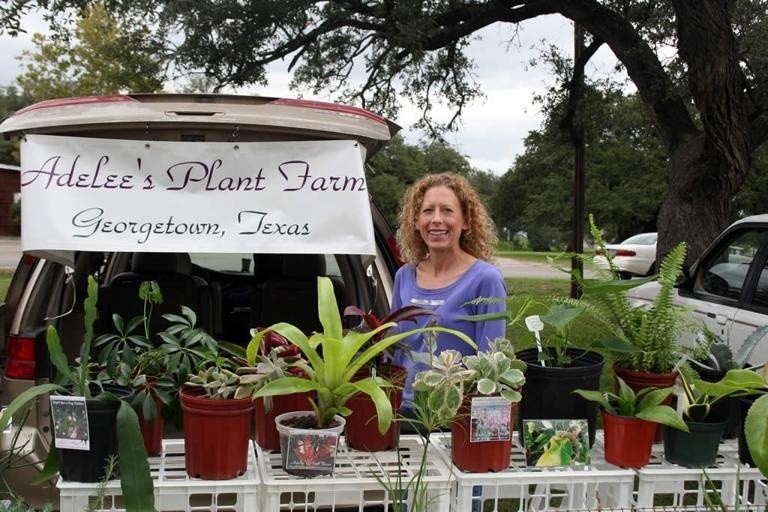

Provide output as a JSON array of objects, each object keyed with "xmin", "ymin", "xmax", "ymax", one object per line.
[{"xmin": 0, "ymin": 94, "xmax": 417, "ymax": 508}]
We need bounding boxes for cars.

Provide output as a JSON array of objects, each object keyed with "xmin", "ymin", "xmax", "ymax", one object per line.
[{"xmin": 592, "ymin": 232, "xmax": 658, "ymax": 280}]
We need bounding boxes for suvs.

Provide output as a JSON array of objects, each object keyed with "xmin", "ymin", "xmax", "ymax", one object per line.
[{"xmin": 621, "ymin": 212, "xmax": 768, "ymax": 393}]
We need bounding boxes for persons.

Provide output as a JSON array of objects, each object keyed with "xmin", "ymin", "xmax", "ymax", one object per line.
[{"xmin": 385, "ymin": 170, "xmax": 507, "ymax": 435}]
